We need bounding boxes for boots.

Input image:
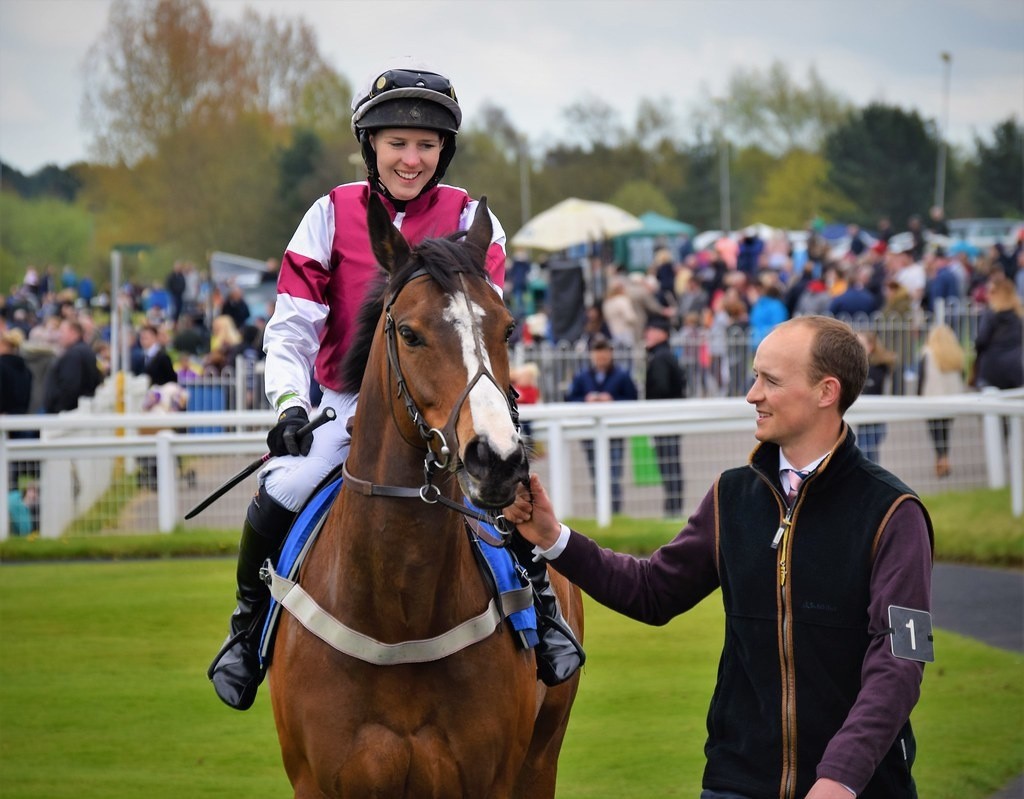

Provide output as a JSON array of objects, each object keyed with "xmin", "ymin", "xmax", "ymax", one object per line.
[
  {"xmin": 490, "ymin": 505, "xmax": 586, "ymax": 686},
  {"xmin": 211, "ymin": 485, "xmax": 300, "ymax": 711}
]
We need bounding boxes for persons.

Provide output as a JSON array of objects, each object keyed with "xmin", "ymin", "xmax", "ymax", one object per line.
[
  {"xmin": 1, "ymin": 197, "xmax": 1024, "ymax": 529},
  {"xmin": 498, "ymin": 311, "xmax": 940, "ymax": 799},
  {"xmin": 207, "ymin": 64, "xmax": 587, "ymax": 706}
]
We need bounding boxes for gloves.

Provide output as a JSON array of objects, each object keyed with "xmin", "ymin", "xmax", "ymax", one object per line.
[{"xmin": 265, "ymin": 405, "xmax": 314, "ymax": 457}]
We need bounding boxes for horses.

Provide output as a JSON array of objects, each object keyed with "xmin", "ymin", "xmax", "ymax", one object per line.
[{"xmin": 267, "ymin": 191, "xmax": 584, "ymax": 799}]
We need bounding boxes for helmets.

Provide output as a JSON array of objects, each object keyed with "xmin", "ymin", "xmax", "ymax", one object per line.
[{"xmin": 349, "ymin": 69, "xmax": 463, "ymax": 142}]
